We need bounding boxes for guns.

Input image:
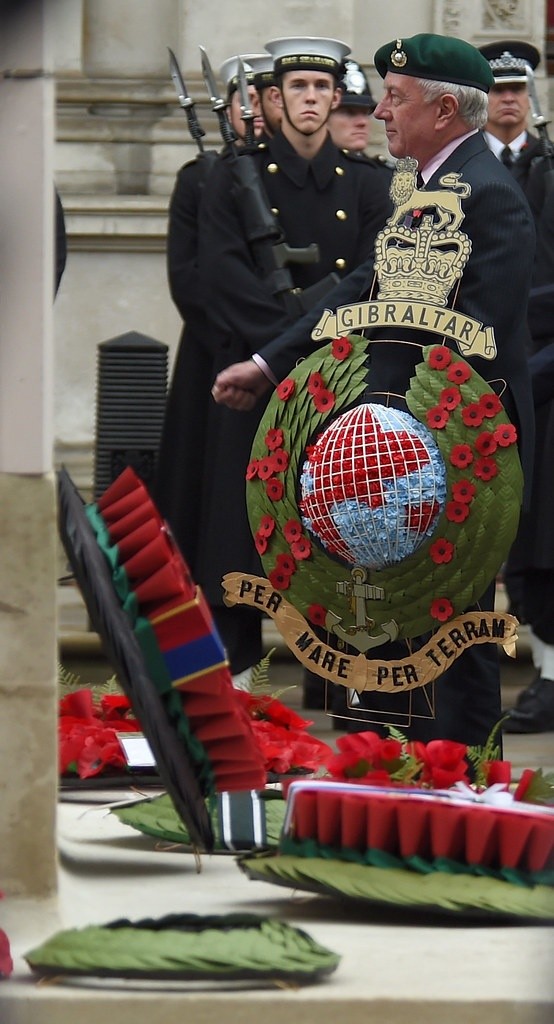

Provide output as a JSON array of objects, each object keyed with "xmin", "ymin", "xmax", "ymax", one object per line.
[
  {"xmin": 524, "ymin": 65, "xmax": 553, "ymax": 159},
  {"xmin": 236, "ymin": 54, "xmax": 262, "ymax": 146},
  {"xmin": 198, "ymin": 44, "xmax": 341, "ymax": 322},
  {"xmin": 166, "ymin": 46, "xmax": 218, "ymax": 157}
]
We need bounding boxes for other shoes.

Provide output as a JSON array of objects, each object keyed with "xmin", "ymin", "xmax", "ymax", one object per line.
[{"xmin": 501, "ymin": 676, "xmax": 554, "ymax": 734}]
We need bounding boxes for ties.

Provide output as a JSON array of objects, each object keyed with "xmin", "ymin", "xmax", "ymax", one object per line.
[{"xmin": 501, "ymin": 146, "xmax": 515, "ymax": 169}]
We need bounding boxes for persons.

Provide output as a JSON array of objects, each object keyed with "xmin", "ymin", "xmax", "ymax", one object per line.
[
  {"xmin": 54, "ymin": 184, "xmax": 69, "ymax": 304},
  {"xmin": 150, "ymin": 33, "xmax": 554, "ymax": 781}
]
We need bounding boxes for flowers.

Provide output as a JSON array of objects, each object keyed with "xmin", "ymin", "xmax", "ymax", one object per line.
[
  {"xmin": 48, "ymin": 647, "xmax": 336, "ymax": 781},
  {"xmin": 324, "ymin": 714, "xmax": 554, "ymax": 809}
]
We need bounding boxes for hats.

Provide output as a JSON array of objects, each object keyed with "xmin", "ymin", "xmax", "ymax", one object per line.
[
  {"xmin": 478, "ymin": 40, "xmax": 541, "ymax": 84},
  {"xmin": 374, "ymin": 32, "xmax": 495, "ymax": 95},
  {"xmin": 264, "ymin": 37, "xmax": 351, "ymax": 78},
  {"xmin": 335, "ymin": 58, "xmax": 378, "ymax": 116},
  {"xmin": 242, "ymin": 55, "xmax": 274, "ymax": 89},
  {"xmin": 220, "ymin": 54, "xmax": 254, "ymax": 103}
]
